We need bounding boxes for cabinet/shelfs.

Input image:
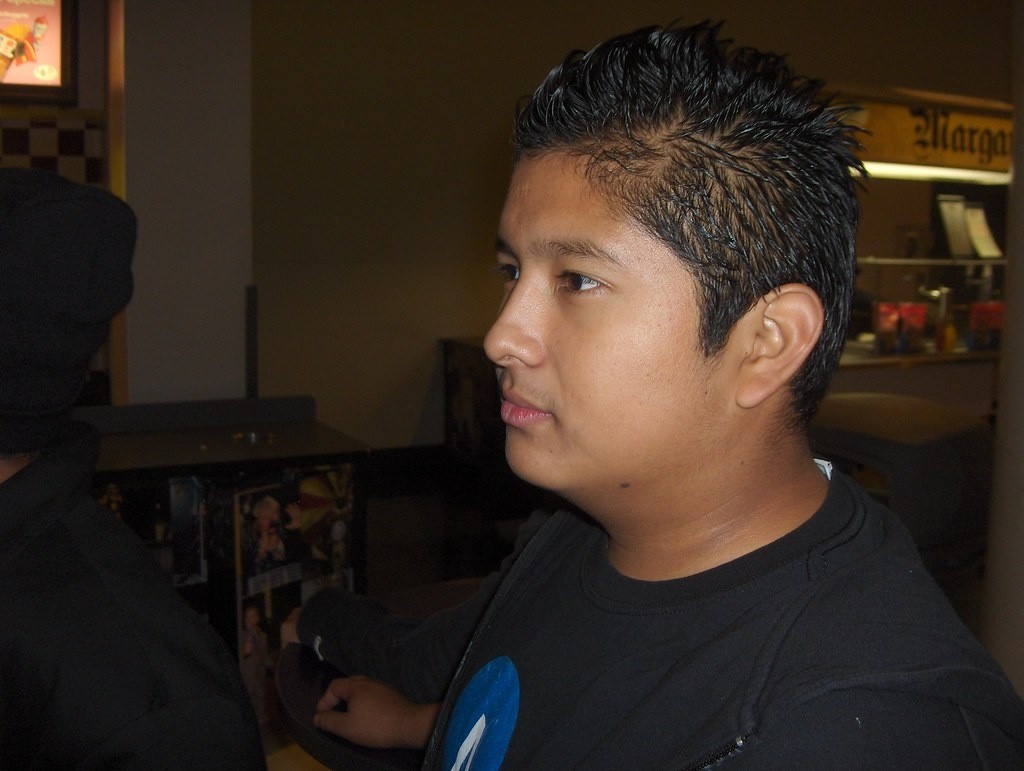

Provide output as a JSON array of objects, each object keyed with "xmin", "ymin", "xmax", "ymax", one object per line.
[
  {"xmin": 90, "ymin": 419, "xmax": 369, "ymax": 755},
  {"xmin": 805, "ymin": 82, "xmax": 1020, "ymax": 424}
]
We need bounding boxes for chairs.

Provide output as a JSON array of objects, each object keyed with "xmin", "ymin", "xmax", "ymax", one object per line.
[{"xmin": 442, "ymin": 340, "xmax": 539, "ymax": 566}]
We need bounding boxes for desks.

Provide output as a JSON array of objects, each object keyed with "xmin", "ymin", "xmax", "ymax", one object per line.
[{"xmin": 272, "ymin": 577, "xmax": 483, "ymax": 771}]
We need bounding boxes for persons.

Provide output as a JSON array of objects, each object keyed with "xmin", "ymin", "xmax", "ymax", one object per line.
[
  {"xmin": 0, "ymin": 166, "xmax": 268, "ymax": 771},
  {"xmin": 281, "ymin": 18, "xmax": 1024, "ymax": 771},
  {"xmin": 239, "ymin": 491, "xmax": 306, "ymax": 726}
]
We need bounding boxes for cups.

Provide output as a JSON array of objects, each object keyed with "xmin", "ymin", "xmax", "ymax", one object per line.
[
  {"xmin": 876, "ymin": 301, "xmax": 898, "ymax": 354},
  {"xmin": 898, "ymin": 301, "xmax": 928, "ymax": 353}
]
